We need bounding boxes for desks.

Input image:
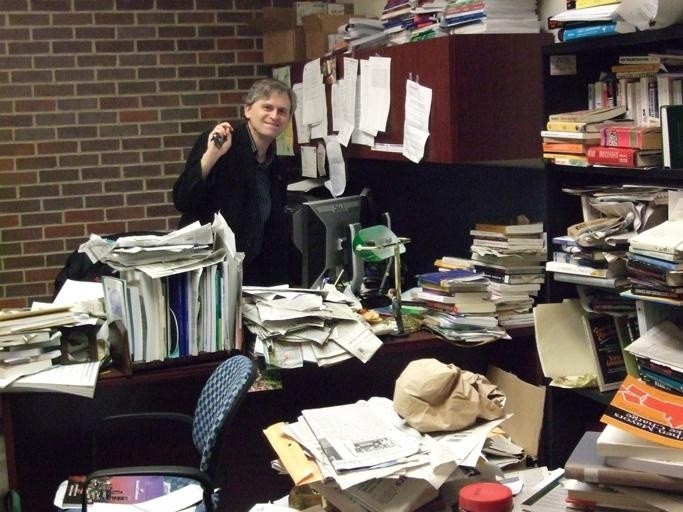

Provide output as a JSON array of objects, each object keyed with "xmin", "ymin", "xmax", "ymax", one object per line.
[{"xmin": 1, "ymin": 294, "xmax": 548, "ymax": 512}]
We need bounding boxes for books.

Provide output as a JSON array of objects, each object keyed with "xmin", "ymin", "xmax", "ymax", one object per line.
[
  {"xmin": 397, "ymin": 210, "xmax": 543, "ymax": 347},
  {"xmin": 0, "ymin": 210, "xmax": 245, "ymax": 399},
  {"xmin": 329, "ymin": 1, "xmax": 540, "ymax": 56},
  {"xmin": 549, "ymin": 0, "xmax": 682, "ymax": 40},
  {"xmin": 258, "ymin": 362, "xmax": 541, "ymax": 512},
  {"xmin": 241, "ymin": 279, "xmax": 384, "ymax": 368},
  {"xmin": 532, "ymin": 184, "xmax": 682, "ymax": 511},
  {"xmin": 541, "ymin": 53, "xmax": 683, "ymax": 170}
]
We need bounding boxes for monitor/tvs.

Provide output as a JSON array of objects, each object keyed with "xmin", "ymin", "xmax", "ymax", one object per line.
[
  {"xmin": 360, "ymin": 188, "xmax": 379, "ymax": 272},
  {"xmin": 302, "ymin": 195, "xmax": 364, "ymax": 297}
]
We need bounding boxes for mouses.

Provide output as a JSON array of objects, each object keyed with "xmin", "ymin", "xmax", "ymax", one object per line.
[{"xmin": 363, "ymin": 278, "xmax": 380, "ymax": 288}]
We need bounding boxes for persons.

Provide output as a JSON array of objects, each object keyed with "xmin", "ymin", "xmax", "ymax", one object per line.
[
  {"xmin": 171, "ymin": 79, "xmax": 303, "ymax": 288},
  {"xmin": 386, "ymin": 288, "xmax": 405, "ymax": 334}
]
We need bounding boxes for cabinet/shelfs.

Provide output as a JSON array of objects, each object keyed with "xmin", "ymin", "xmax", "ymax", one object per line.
[
  {"xmin": 536, "ymin": 19, "xmax": 682, "ymax": 473},
  {"xmin": 267, "ymin": 35, "xmax": 546, "ymax": 166}
]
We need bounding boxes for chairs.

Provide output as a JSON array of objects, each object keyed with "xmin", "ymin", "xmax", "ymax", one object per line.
[{"xmin": 51, "ymin": 349, "xmax": 259, "ymax": 510}]
